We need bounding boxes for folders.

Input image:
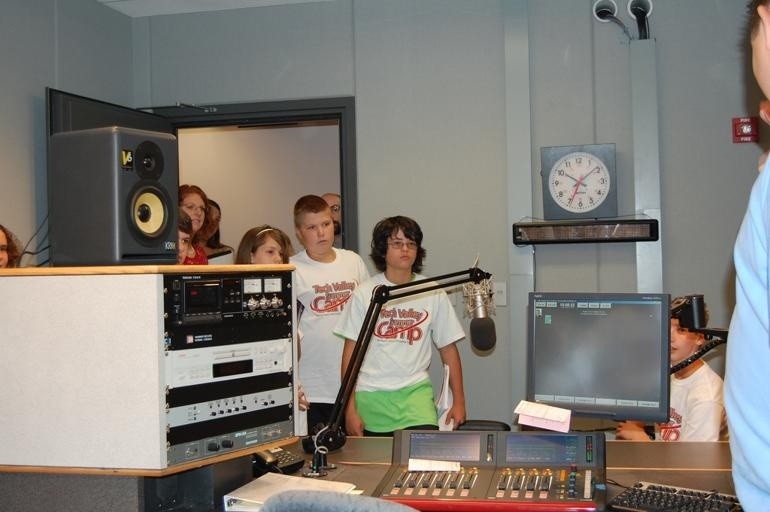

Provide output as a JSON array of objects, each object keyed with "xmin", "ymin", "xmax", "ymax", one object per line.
[{"xmin": 224, "ymin": 472, "xmax": 364, "ymax": 512}]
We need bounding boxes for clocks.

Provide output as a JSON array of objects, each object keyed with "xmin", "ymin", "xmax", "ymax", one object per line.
[{"xmin": 540, "ymin": 142, "xmax": 619, "ymax": 220}]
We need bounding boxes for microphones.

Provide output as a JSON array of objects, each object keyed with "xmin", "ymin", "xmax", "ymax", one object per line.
[{"xmin": 468, "ymin": 279, "xmax": 498, "ymax": 356}]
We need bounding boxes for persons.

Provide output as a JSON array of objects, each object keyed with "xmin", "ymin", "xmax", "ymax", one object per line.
[
  {"xmin": 288, "ymin": 193, "xmax": 370, "ymax": 432},
  {"xmin": 329, "ymin": 215, "xmax": 467, "ymax": 438},
  {"xmin": 322, "ymin": 193, "xmax": 343, "ymax": 248},
  {"xmin": 177, "ymin": 208, "xmax": 193, "ymax": 264},
  {"xmin": 234, "ymin": 225, "xmax": 289, "ymax": 264},
  {"xmin": 197, "ymin": 199, "xmax": 235, "ymax": 265},
  {"xmin": 616, "ymin": 296, "xmax": 728, "ymax": 442},
  {"xmin": 721, "ymin": 0, "xmax": 769, "ymax": 512},
  {"xmin": 0, "ymin": 224, "xmax": 19, "ymax": 269},
  {"xmin": 179, "ymin": 184, "xmax": 208, "ymax": 265}
]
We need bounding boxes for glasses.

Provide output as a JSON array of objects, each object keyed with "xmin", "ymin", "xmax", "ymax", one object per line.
[
  {"xmin": 182, "ymin": 203, "xmax": 206, "ymax": 212},
  {"xmin": 388, "ymin": 241, "xmax": 417, "ymax": 249}
]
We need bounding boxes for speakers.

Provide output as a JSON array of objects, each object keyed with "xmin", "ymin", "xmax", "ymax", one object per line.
[{"xmin": 48, "ymin": 127, "xmax": 178, "ymax": 270}]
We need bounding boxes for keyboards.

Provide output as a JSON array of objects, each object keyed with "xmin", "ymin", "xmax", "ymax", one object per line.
[{"xmin": 609, "ymin": 480, "xmax": 743, "ymax": 511}]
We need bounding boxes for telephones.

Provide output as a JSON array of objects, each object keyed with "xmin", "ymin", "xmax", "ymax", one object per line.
[{"xmin": 252, "ymin": 447, "xmax": 305, "ymax": 478}]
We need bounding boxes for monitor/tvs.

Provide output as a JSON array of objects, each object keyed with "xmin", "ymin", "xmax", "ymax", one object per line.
[{"xmin": 528, "ymin": 289, "xmax": 672, "ymax": 428}]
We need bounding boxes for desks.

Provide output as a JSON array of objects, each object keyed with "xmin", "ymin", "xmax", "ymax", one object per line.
[
  {"xmin": 0, "ymin": 436, "xmax": 298, "ymax": 512},
  {"xmin": 281, "ymin": 435, "xmax": 734, "ymax": 503}
]
